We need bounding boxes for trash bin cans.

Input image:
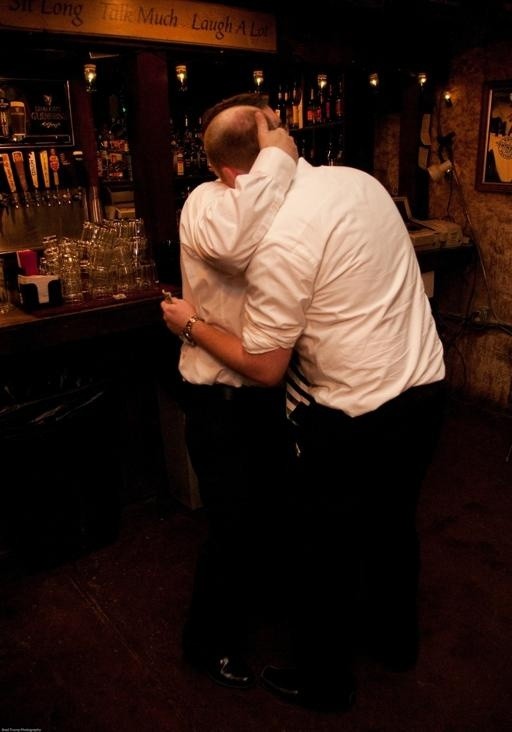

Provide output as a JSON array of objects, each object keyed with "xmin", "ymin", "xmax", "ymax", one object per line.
[{"xmin": 35, "ymin": 393, "xmax": 121, "ymax": 551}]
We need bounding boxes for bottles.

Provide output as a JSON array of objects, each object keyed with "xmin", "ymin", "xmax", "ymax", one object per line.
[
  {"xmin": 299, "ymin": 135, "xmax": 345, "ymax": 167},
  {"xmin": 275, "ymin": 79, "xmax": 345, "ymax": 130},
  {"xmin": 166, "ymin": 114, "xmax": 206, "ymax": 175}
]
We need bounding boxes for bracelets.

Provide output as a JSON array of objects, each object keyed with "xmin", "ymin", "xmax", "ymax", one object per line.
[{"xmin": 177, "ymin": 312, "xmax": 205, "ymax": 346}]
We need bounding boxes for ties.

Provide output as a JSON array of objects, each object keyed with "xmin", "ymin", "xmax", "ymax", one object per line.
[{"xmin": 284, "ymin": 352, "xmax": 310, "ymax": 424}]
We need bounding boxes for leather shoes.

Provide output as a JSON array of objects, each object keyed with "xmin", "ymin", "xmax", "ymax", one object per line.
[
  {"xmin": 211, "ymin": 652, "xmax": 275, "ymax": 690},
  {"xmin": 259, "ymin": 665, "xmax": 301, "ymax": 704}
]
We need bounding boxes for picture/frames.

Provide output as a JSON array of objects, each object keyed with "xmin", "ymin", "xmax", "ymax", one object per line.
[{"xmin": 475, "ymin": 78, "xmax": 512, "ymax": 194}]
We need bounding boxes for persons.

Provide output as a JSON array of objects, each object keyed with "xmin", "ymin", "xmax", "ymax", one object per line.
[
  {"xmin": 177, "ymin": 93, "xmax": 290, "ymax": 691},
  {"xmin": 157, "ymin": 101, "xmax": 450, "ymax": 718}
]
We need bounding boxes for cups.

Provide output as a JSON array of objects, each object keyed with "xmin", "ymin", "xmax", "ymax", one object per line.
[{"xmin": 42, "ymin": 215, "xmax": 155, "ymax": 306}]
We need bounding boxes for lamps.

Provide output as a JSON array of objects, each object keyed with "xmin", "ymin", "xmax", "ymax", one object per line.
[
  {"xmin": 176, "ymin": 64, "xmax": 188, "ymax": 92},
  {"xmin": 83, "ymin": 63, "xmax": 97, "ymax": 92},
  {"xmin": 418, "ymin": 72, "xmax": 427, "ymax": 90},
  {"xmin": 318, "ymin": 73, "xmax": 329, "ymax": 92},
  {"xmin": 252, "ymin": 70, "xmax": 264, "ymax": 90},
  {"xmin": 443, "ymin": 91, "xmax": 453, "ymax": 107},
  {"xmin": 368, "ymin": 72, "xmax": 379, "ymax": 87}
]
